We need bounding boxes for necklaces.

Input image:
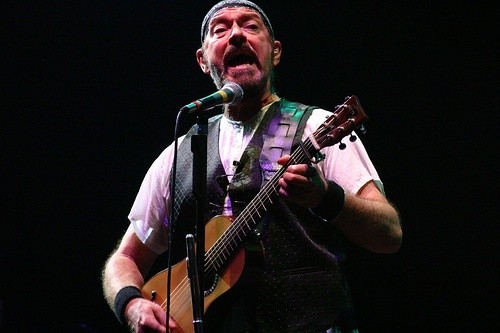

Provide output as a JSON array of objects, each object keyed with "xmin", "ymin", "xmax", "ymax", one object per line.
[{"xmin": 261, "ymin": 92, "xmax": 273, "ymax": 103}]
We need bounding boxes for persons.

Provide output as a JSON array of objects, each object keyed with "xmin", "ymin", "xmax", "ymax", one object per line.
[{"xmin": 101, "ymin": 0, "xmax": 402, "ymax": 333}]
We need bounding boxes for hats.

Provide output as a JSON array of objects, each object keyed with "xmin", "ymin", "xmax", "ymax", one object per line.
[{"xmin": 201, "ymin": 0, "xmax": 274, "ymax": 42}]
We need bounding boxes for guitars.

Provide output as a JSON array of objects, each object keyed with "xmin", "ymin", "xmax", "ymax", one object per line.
[{"xmin": 141, "ymin": 95, "xmax": 370, "ymax": 333}]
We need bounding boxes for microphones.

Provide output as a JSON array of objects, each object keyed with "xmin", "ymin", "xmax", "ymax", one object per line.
[{"xmin": 181, "ymin": 82, "xmax": 245, "ymax": 114}]
